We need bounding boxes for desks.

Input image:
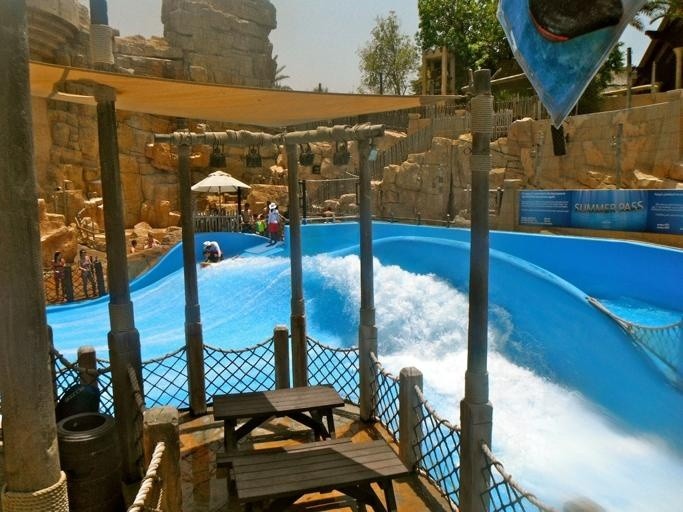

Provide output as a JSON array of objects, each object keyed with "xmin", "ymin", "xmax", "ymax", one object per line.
[{"xmin": 212, "ymin": 383, "xmax": 410, "ymax": 512}]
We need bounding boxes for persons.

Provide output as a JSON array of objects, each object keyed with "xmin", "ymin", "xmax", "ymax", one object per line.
[
  {"xmin": 143, "ymin": 230, "xmax": 161, "ymax": 248},
  {"xmin": 202, "ymin": 240, "xmax": 222, "ymax": 262},
  {"xmin": 50, "ymin": 250, "xmax": 66, "ymax": 302},
  {"xmin": 130, "ymin": 240, "xmax": 139, "ymax": 254},
  {"xmin": 76, "ymin": 248, "xmax": 97, "ymax": 299},
  {"xmin": 202, "ymin": 251, "xmax": 223, "ymax": 263},
  {"xmin": 320, "ymin": 206, "xmax": 340, "ymax": 223},
  {"xmin": 197, "ymin": 200, "xmax": 285, "ymax": 245}
]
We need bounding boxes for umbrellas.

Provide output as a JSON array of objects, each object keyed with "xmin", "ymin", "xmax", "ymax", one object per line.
[{"xmin": 190, "ymin": 170, "xmax": 253, "ymax": 208}]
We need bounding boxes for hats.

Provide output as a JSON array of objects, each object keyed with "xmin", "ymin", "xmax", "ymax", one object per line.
[{"xmin": 268, "ymin": 202, "xmax": 277, "ymax": 209}]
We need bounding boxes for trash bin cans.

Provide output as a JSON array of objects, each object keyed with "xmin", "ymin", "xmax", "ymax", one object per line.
[{"xmin": 56, "ymin": 412, "xmax": 123, "ymax": 511}]
